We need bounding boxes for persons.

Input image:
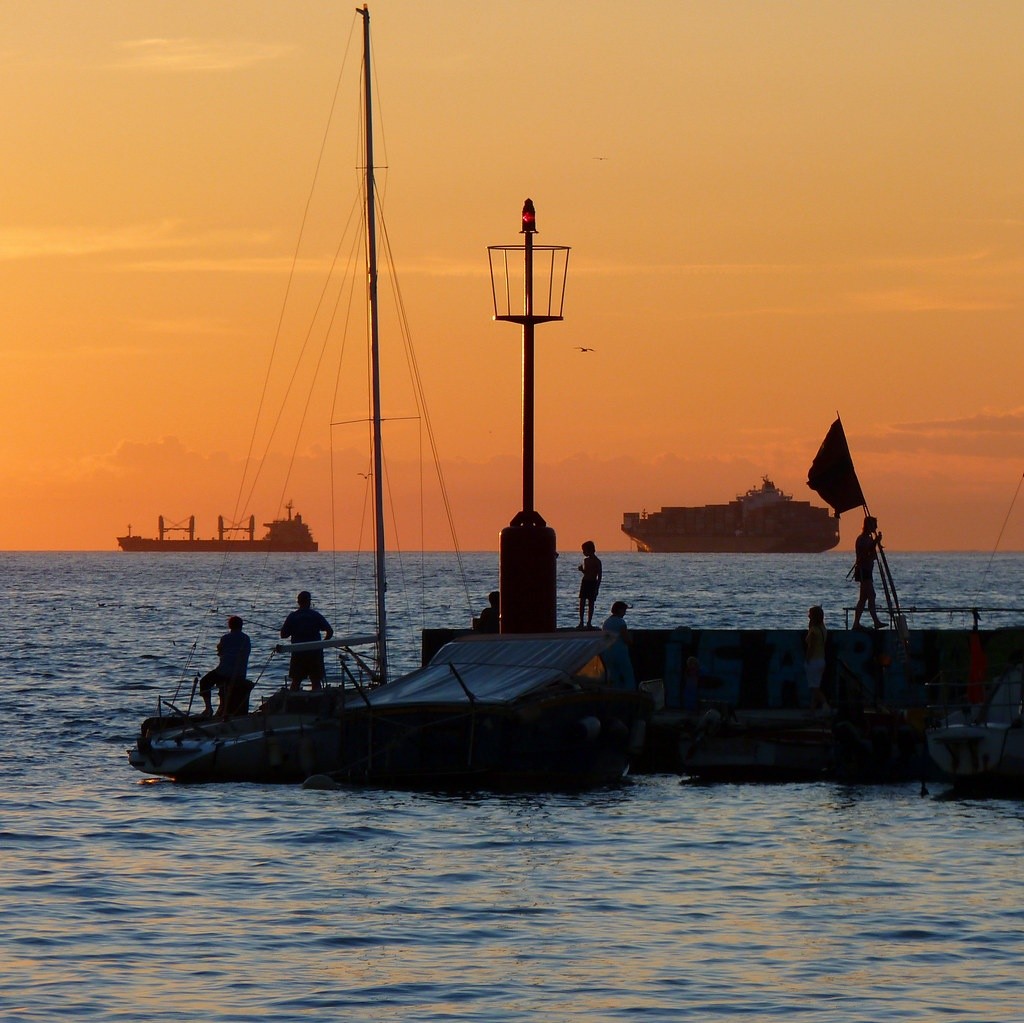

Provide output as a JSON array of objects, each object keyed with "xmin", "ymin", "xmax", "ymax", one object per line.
[
  {"xmin": 281, "ymin": 591, "xmax": 333, "ymax": 690},
  {"xmin": 601, "ymin": 601, "xmax": 633, "ymax": 688},
  {"xmin": 576, "ymin": 541, "xmax": 602, "ymax": 630},
  {"xmin": 853, "ymin": 517, "xmax": 890, "ymax": 629},
  {"xmin": 479, "ymin": 591, "xmax": 499, "ymax": 633},
  {"xmin": 199, "ymin": 617, "xmax": 251, "ymax": 718},
  {"xmin": 801, "ymin": 607, "xmax": 833, "ymax": 717}
]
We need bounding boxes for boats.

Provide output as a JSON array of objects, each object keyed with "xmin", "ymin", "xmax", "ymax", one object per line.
[
  {"xmin": 620, "ymin": 474, "xmax": 843, "ymax": 553},
  {"xmin": 344, "ymin": 624, "xmax": 662, "ymax": 806},
  {"xmin": 919, "ymin": 647, "xmax": 1024, "ymax": 801},
  {"xmin": 116, "ymin": 498, "xmax": 319, "ymax": 552}
]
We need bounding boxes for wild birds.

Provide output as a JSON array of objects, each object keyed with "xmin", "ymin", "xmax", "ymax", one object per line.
[
  {"xmin": 573, "ymin": 347, "xmax": 594, "ymax": 352},
  {"xmin": 356, "ymin": 473, "xmax": 373, "ymax": 478}
]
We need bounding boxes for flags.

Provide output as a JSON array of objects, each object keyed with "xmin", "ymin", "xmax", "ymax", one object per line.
[{"xmin": 806, "ymin": 418, "xmax": 865, "ymax": 519}]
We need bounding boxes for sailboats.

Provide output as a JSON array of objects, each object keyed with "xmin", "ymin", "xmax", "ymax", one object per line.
[{"xmin": 125, "ymin": 5, "xmax": 401, "ymax": 784}]
